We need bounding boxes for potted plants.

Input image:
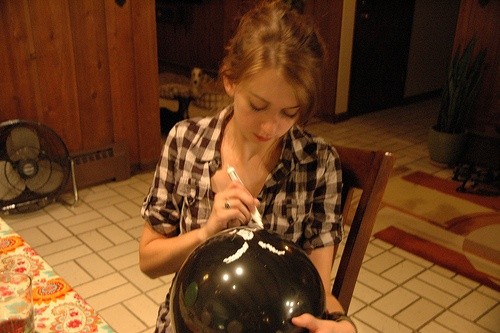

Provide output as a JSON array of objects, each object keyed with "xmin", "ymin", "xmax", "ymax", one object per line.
[{"xmin": 428, "ymin": 35, "xmax": 489, "ymax": 164}]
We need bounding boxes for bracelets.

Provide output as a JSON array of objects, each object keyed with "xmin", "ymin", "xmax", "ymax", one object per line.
[{"xmin": 328, "ymin": 313, "xmax": 358, "ymax": 333}]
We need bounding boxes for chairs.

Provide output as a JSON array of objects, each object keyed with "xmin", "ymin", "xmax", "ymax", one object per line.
[{"xmin": 323, "ymin": 144, "xmax": 396, "ymax": 313}]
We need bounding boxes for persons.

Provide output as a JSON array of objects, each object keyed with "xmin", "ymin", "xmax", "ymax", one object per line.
[{"xmin": 139, "ymin": 4, "xmax": 358, "ymax": 333}]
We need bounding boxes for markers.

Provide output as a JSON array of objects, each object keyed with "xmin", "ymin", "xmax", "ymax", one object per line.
[{"xmin": 226, "ymin": 164, "xmax": 264, "ymax": 229}]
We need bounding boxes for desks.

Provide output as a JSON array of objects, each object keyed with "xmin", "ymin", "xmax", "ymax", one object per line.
[{"xmin": 0, "ymin": 215, "xmax": 117, "ymax": 333}]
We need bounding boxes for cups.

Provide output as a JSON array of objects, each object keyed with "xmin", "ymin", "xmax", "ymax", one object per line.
[{"xmin": 0, "ymin": 272, "xmax": 36, "ymax": 333}]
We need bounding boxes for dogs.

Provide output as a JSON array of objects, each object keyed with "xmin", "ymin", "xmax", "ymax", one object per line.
[{"xmin": 159, "ymin": 67, "xmax": 211, "ymax": 112}]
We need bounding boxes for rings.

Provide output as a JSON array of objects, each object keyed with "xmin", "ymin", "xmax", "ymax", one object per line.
[{"xmin": 225, "ymin": 200, "xmax": 230, "ymax": 209}]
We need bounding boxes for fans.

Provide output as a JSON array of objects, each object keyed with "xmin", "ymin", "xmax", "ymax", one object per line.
[{"xmin": 0, "ymin": 119, "xmax": 72, "ymax": 216}]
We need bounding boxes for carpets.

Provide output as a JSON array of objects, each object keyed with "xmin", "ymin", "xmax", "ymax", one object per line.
[{"xmin": 342, "ymin": 147, "xmax": 500, "ymax": 291}]
